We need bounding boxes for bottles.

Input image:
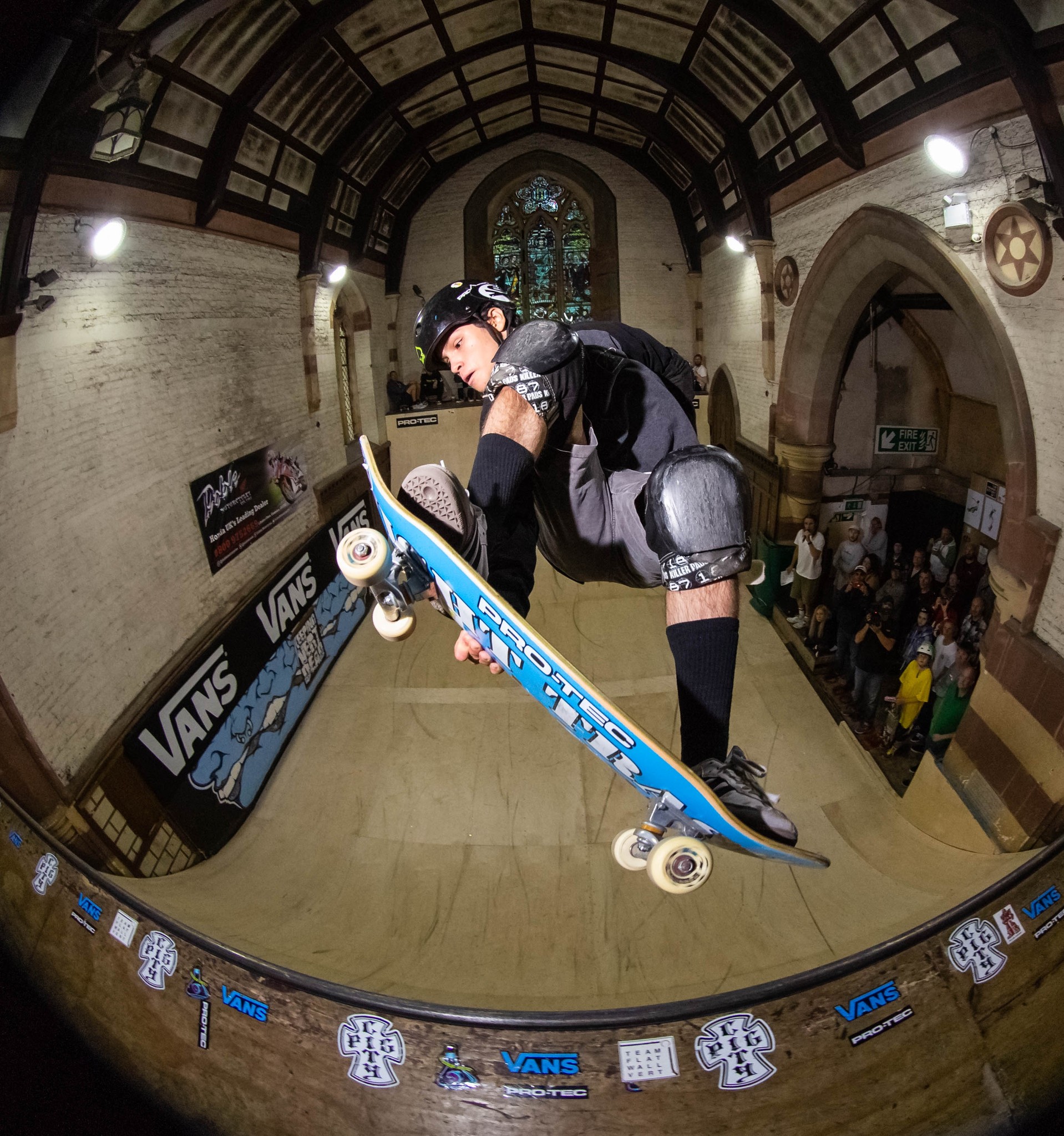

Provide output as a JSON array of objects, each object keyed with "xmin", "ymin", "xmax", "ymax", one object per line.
[{"xmin": 884, "ymin": 696, "xmax": 896, "ymax": 702}]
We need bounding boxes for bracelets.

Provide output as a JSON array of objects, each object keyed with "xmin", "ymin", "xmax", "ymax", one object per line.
[{"xmin": 808, "ymin": 542, "xmax": 812, "ymax": 545}]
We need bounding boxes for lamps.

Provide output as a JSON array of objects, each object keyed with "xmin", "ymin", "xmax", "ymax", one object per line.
[
  {"xmin": 725, "ymin": 235, "xmax": 753, "ymax": 253},
  {"xmin": 943, "ymin": 193, "xmax": 971, "ymax": 229},
  {"xmin": 318, "ymin": 262, "xmax": 347, "ymax": 287},
  {"xmin": 90, "ymin": 67, "xmax": 150, "ymax": 163},
  {"xmin": 75, "ymin": 216, "xmax": 127, "ymax": 267},
  {"xmin": 924, "ymin": 124, "xmax": 995, "ymax": 178},
  {"xmin": 655, "ymin": 263, "xmax": 687, "ymax": 271}
]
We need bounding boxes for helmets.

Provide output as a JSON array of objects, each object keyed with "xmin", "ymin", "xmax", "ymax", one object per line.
[
  {"xmin": 916, "ymin": 643, "xmax": 935, "ymax": 659},
  {"xmin": 412, "ymin": 280, "xmax": 517, "ymax": 372}
]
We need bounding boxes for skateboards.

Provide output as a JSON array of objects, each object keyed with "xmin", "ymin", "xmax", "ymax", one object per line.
[
  {"xmin": 335, "ymin": 432, "xmax": 831, "ymax": 898},
  {"xmin": 877, "ymin": 702, "xmax": 902, "ymax": 752}
]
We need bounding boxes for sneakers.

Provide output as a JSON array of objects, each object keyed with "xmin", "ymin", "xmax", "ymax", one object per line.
[
  {"xmin": 689, "ymin": 745, "xmax": 798, "ymax": 844},
  {"xmin": 792, "ymin": 620, "xmax": 809, "ymax": 628},
  {"xmin": 423, "ymin": 402, "xmax": 428, "ymax": 405},
  {"xmin": 456, "ymin": 400, "xmax": 464, "ymax": 403},
  {"xmin": 468, "ymin": 398, "xmax": 474, "ymax": 401},
  {"xmin": 786, "ymin": 614, "xmax": 804, "ymax": 623},
  {"xmin": 397, "ymin": 460, "xmax": 489, "ymax": 582},
  {"xmin": 436, "ymin": 401, "xmax": 442, "ymax": 404}
]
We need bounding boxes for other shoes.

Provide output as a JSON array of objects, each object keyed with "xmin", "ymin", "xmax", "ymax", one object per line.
[
  {"xmin": 909, "ymin": 766, "xmax": 919, "ymax": 773},
  {"xmin": 902, "ymin": 779, "xmax": 912, "ymax": 786},
  {"xmin": 910, "ymin": 747, "xmax": 925, "ymax": 755},
  {"xmin": 910, "ymin": 733, "xmax": 922, "ymax": 741},
  {"xmin": 822, "ymin": 674, "xmax": 898, "ymax": 760}
]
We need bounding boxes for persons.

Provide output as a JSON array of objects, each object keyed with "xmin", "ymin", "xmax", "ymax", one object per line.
[
  {"xmin": 454, "ymin": 375, "xmax": 475, "ymax": 403},
  {"xmin": 867, "ymin": 517, "xmax": 888, "ymax": 567},
  {"xmin": 387, "ymin": 370, "xmax": 428, "ymax": 409},
  {"xmin": 787, "ymin": 514, "xmax": 826, "ymax": 629},
  {"xmin": 830, "ymin": 523, "xmax": 989, "ymax": 787},
  {"xmin": 832, "ymin": 525, "xmax": 865, "ymax": 591},
  {"xmin": 803, "ymin": 604, "xmax": 833, "ymax": 657},
  {"xmin": 840, "ymin": 595, "xmax": 899, "ymax": 734},
  {"xmin": 396, "ymin": 279, "xmax": 799, "ymax": 852},
  {"xmin": 421, "ymin": 369, "xmax": 444, "ymax": 405}
]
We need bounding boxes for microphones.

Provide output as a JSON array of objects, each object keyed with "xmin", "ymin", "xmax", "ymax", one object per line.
[{"xmin": 803, "ymin": 529, "xmax": 810, "ymax": 542}]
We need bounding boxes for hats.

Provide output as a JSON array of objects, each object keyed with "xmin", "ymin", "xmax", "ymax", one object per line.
[
  {"xmin": 849, "ymin": 525, "xmax": 859, "ymax": 531},
  {"xmin": 892, "ymin": 562, "xmax": 903, "ymax": 570},
  {"xmin": 854, "ymin": 566, "xmax": 867, "ymax": 573}
]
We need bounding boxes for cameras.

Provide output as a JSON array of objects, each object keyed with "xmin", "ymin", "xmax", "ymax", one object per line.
[
  {"xmin": 852, "ymin": 582, "xmax": 862, "ymax": 588},
  {"xmin": 940, "ymin": 596, "xmax": 948, "ymax": 606}
]
